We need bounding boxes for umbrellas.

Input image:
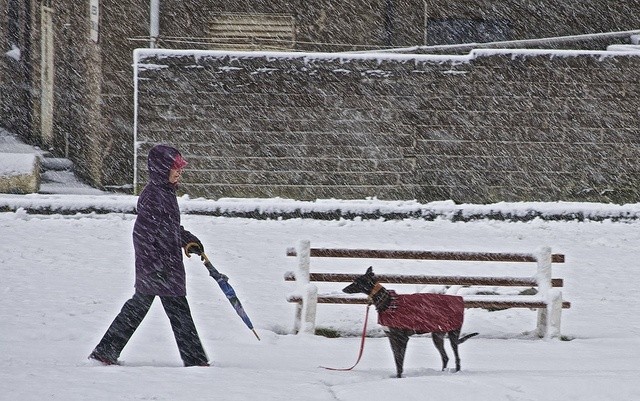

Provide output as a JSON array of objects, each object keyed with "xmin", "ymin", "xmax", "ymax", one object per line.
[{"xmin": 184, "ymin": 243, "xmax": 260, "ymax": 341}]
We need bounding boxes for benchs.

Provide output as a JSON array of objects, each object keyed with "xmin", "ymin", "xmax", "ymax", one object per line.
[{"xmin": 284, "ymin": 241, "xmax": 571, "ymax": 339}]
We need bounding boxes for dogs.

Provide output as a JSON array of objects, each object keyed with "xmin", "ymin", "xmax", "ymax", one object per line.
[{"xmin": 342, "ymin": 267, "xmax": 480, "ymax": 378}]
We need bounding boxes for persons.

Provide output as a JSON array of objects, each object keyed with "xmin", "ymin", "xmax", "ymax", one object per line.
[{"xmin": 88, "ymin": 144, "xmax": 213, "ymax": 368}]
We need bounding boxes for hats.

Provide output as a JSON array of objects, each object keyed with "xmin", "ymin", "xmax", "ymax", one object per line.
[{"xmin": 169, "ymin": 152, "xmax": 189, "ymax": 169}]
